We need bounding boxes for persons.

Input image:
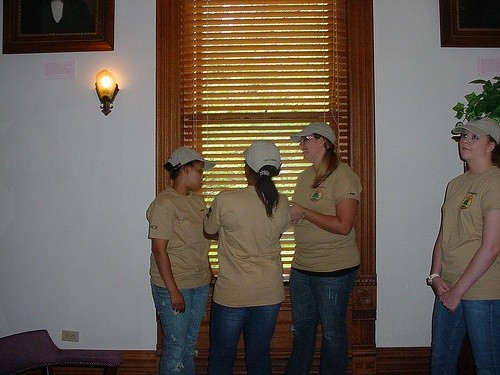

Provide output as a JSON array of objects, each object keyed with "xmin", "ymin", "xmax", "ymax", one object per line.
[
  {"xmin": 146, "ymin": 147, "xmax": 217, "ymax": 375},
  {"xmin": 202, "ymin": 140, "xmax": 291, "ymax": 375},
  {"xmin": 284, "ymin": 121, "xmax": 362, "ymax": 375},
  {"xmin": 426, "ymin": 116, "xmax": 500, "ymax": 375}
]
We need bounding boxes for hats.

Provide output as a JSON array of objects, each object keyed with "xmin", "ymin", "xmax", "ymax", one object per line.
[
  {"xmin": 452, "ymin": 117, "xmax": 500, "ymax": 145},
  {"xmin": 168, "ymin": 148, "xmax": 216, "ymax": 170},
  {"xmin": 243, "ymin": 141, "xmax": 280, "ymax": 172},
  {"xmin": 291, "ymin": 122, "xmax": 336, "ymax": 144}
]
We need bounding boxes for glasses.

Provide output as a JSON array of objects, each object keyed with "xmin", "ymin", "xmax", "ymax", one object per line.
[{"xmin": 300, "ymin": 136, "xmax": 318, "ymax": 142}]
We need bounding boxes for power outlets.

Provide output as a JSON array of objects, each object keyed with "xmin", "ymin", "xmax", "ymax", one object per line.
[{"xmin": 62, "ymin": 330, "xmax": 79, "ymax": 341}]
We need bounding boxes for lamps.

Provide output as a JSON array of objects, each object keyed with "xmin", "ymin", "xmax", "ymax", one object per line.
[{"xmin": 94, "ymin": 69, "xmax": 118, "ymax": 114}]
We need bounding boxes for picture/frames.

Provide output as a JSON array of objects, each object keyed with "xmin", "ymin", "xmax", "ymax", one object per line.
[
  {"xmin": 3, "ymin": 0, "xmax": 115, "ymax": 54},
  {"xmin": 439, "ymin": 0, "xmax": 500, "ymax": 48}
]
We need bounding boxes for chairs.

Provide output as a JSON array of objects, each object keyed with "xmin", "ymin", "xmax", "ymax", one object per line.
[{"xmin": 0, "ymin": 330, "xmax": 121, "ymax": 375}]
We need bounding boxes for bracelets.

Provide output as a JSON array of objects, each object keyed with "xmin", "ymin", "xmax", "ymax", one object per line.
[
  {"xmin": 426, "ymin": 274, "xmax": 440, "ymax": 285},
  {"xmin": 302, "ymin": 208, "xmax": 305, "ymax": 219}
]
